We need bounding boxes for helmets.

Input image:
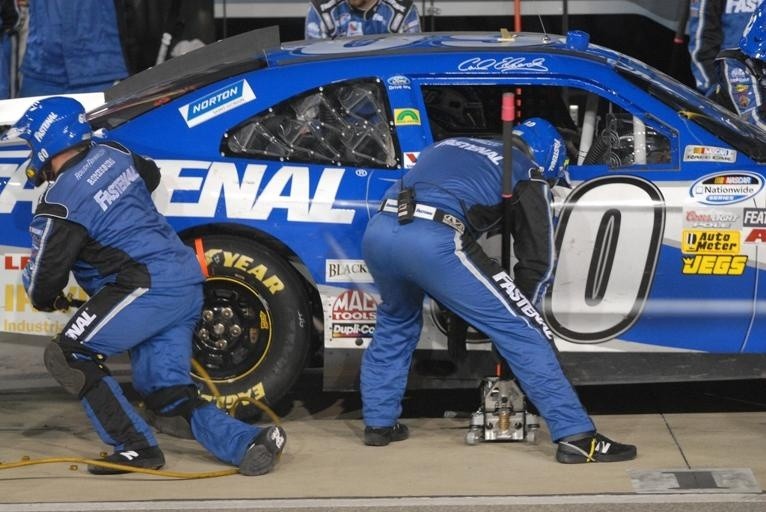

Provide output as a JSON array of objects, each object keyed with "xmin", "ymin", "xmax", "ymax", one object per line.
[
  {"xmin": 509, "ymin": 116, "xmax": 570, "ymax": 182},
  {"xmin": 737, "ymin": 0, "xmax": 766, "ymax": 63},
  {"xmin": 0, "ymin": 96, "xmax": 94, "ymax": 188}
]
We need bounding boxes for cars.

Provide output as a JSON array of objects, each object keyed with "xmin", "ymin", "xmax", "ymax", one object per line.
[{"xmin": 1, "ymin": 30, "xmax": 761, "ymax": 420}]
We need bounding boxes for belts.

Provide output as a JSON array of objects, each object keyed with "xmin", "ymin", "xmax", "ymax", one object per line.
[{"xmin": 378, "ymin": 198, "xmax": 469, "ymax": 233}]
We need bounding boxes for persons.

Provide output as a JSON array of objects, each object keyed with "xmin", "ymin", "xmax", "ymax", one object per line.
[
  {"xmin": 360, "ymin": 118, "xmax": 637, "ymax": 464},
  {"xmin": 687, "ymin": 0, "xmax": 759, "ymax": 95},
  {"xmin": 705, "ymin": 1, "xmax": 766, "ymax": 128},
  {"xmin": 303, "ymin": 0, "xmax": 421, "ymax": 40},
  {"xmin": 14, "ymin": 98, "xmax": 286, "ymax": 476}
]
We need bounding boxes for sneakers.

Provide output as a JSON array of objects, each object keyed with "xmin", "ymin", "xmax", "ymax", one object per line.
[
  {"xmin": 555, "ymin": 431, "xmax": 638, "ymax": 465},
  {"xmin": 238, "ymin": 423, "xmax": 289, "ymax": 476},
  {"xmin": 361, "ymin": 421, "xmax": 412, "ymax": 447},
  {"xmin": 88, "ymin": 445, "xmax": 167, "ymax": 476}
]
